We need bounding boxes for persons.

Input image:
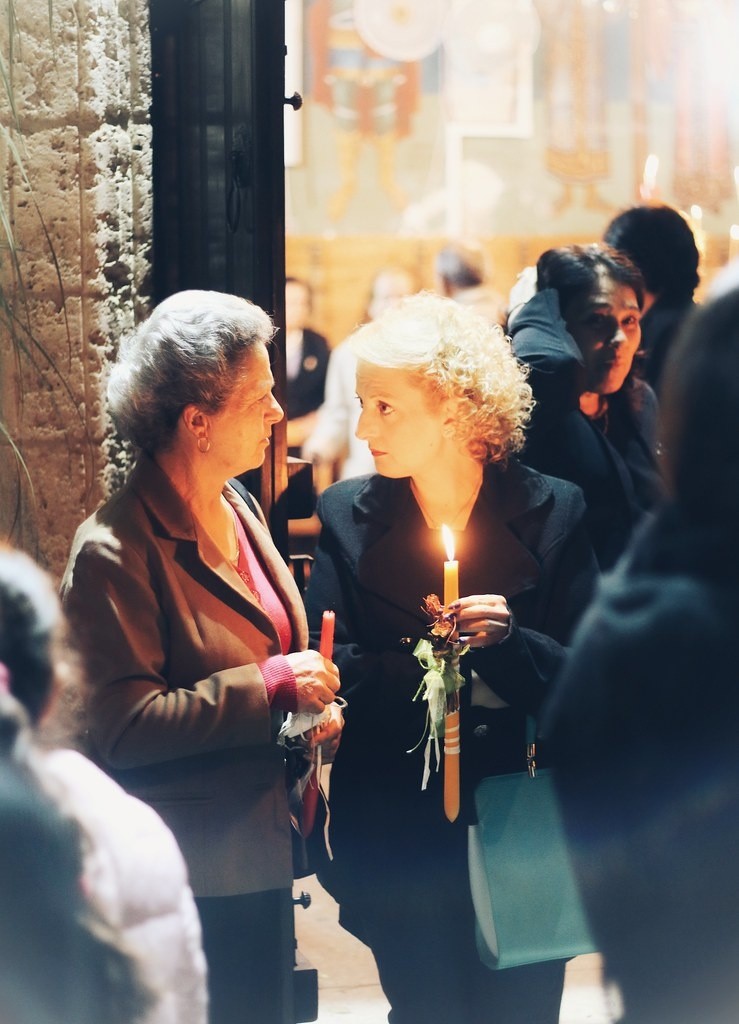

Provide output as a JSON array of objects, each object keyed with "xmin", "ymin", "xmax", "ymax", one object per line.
[
  {"xmin": 538, "ymin": 287, "xmax": 739, "ymax": 1024},
  {"xmin": 603, "ymin": 205, "xmax": 700, "ymax": 386},
  {"xmin": 285, "ymin": 278, "xmax": 331, "ymax": 459},
  {"xmin": 302, "ymin": 269, "xmax": 414, "ymax": 497},
  {"xmin": 58, "ymin": 289, "xmax": 340, "ymax": 1024},
  {"xmin": 510, "ymin": 243, "xmax": 659, "ymax": 573},
  {"xmin": 0, "ymin": 545, "xmax": 210, "ymax": 1024},
  {"xmin": 505, "ymin": 267, "xmax": 539, "ymax": 328},
  {"xmin": 305, "ymin": 293, "xmax": 603, "ymax": 1024}
]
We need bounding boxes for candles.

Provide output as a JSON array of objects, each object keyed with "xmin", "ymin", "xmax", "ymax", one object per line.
[
  {"xmin": 301, "ymin": 608, "xmax": 335, "ymax": 836},
  {"xmin": 442, "ymin": 524, "xmax": 460, "ymax": 822}
]
